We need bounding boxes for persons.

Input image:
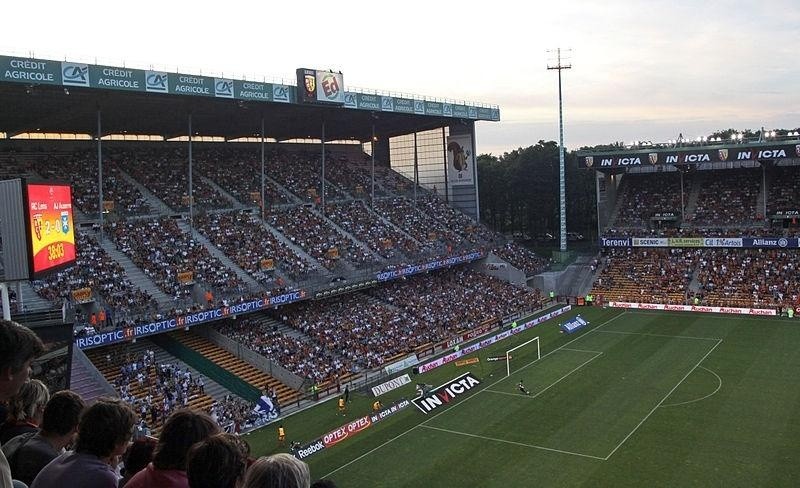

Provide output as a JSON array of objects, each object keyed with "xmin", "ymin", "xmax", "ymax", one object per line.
[
  {"xmin": 27, "ymin": 393, "xmax": 139, "ymax": 487},
  {"xmin": 586, "ymin": 172, "xmax": 800, "ymax": 316},
  {"xmin": 0, "ymin": 138, "xmax": 552, "ymax": 438},
  {"xmin": 241, "ymin": 452, "xmax": 313, "ymax": 488},
  {"xmin": 0, "ymin": 317, "xmax": 50, "ymax": 487},
  {"xmin": 184, "ymin": 431, "xmax": 251, "ymax": 487},
  {"xmin": 549, "ymin": 290, "xmax": 555, "ymax": 301},
  {"xmin": 122, "ymin": 409, "xmax": 226, "ymax": 487},
  {"xmin": 0, "ymin": 385, "xmax": 90, "ymax": 488}
]
